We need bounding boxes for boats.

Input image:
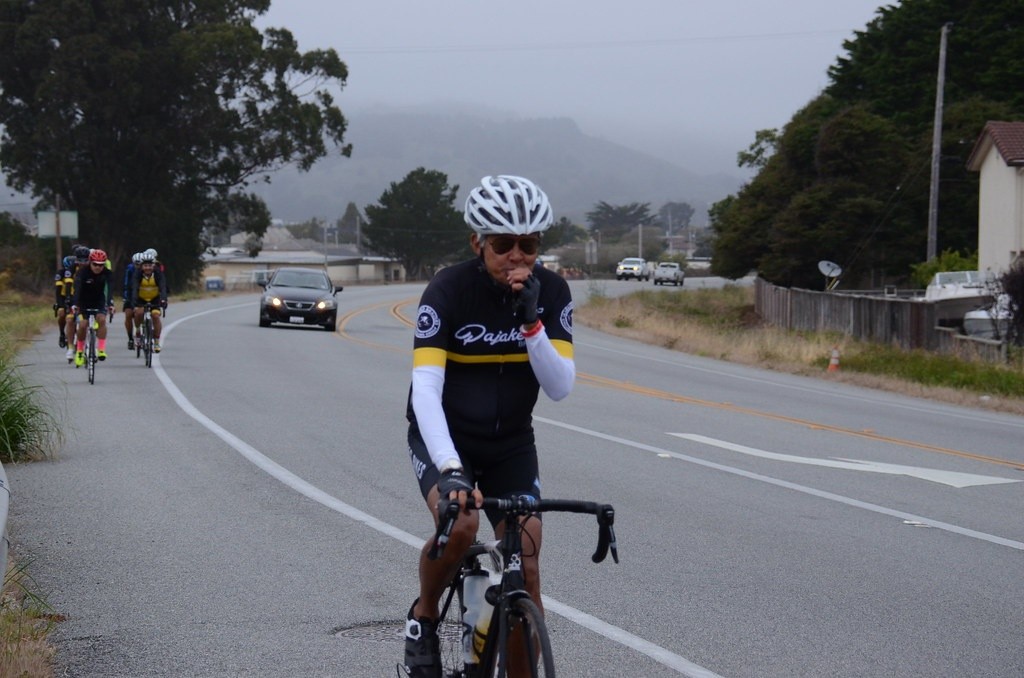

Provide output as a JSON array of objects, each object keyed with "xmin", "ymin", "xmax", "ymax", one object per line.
[{"xmin": 910, "ymin": 269, "xmax": 1018, "ymax": 345}]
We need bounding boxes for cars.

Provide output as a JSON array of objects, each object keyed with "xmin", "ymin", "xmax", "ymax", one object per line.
[
  {"xmin": 257, "ymin": 267, "xmax": 344, "ymax": 332},
  {"xmin": 616, "ymin": 257, "xmax": 651, "ymax": 282},
  {"xmin": 653, "ymin": 263, "xmax": 686, "ymax": 287}
]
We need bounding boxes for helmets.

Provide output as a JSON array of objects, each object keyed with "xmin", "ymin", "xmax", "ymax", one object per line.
[
  {"xmin": 140, "ymin": 253, "xmax": 156, "ymax": 264},
  {"xmin": 463, "ymin": 174, "xmax": 555, "ymax": 248},
  {"xmin": 63, "ymin": 244, "xmax": 94, "ymax": 270},
  {"xmin": 144, "ymin": 248, "xmax": 158, "ymax": 257},
  {"xmin": 132, "ymin": 253, "xmax": 144, "ymax": 264},
  {"xmin": 89, "ymin": 250, "xmax": 107, "ymax": 264}
]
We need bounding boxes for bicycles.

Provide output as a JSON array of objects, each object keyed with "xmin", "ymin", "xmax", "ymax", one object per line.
[
  {"xmin": 129, "ymin": 301, "xmax": 167, "ymax": 368},
  {"xmin": 52, "ymin": 298, "xmax": 141, "ymax": 369},
  {"xmin": 73, "ymin": 307, "xmax": 113, "ymax": 385},
  {"xmin": 395, "ymin": 495, "xmax": 619, "ymax": 678}
]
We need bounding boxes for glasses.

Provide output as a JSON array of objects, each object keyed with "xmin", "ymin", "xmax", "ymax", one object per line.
[
  {"xmin": 93, "ymin": 263, "xmax": 104, "ymax": 267},
  {"xmin": 487, "ymin": 235, "xmax": 542, "ymax": 256}
]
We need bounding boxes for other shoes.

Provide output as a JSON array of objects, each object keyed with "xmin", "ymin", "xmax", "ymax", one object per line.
[
  {"xmin": 154, "ymin": 344, "xmax": 161, "ymax": 352},
  {"xmin": 127, "ymin": 339, "xmax": 134, "ymax": 350},
  {"xmin": 59, "ymin": 333, "xmax": 65, "ymax": 348},
  {"xmin": 134, "ymin": 337, "xmax": 142, "ymax": 347},
  {"xmin": 66, "ymin": 345, "xmax": 75, "ymax": 360}
]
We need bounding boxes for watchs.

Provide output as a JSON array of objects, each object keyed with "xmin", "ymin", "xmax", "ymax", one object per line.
[{"xmin": 440, "ymin": 459, "xmax": 464, "ymax": 472}]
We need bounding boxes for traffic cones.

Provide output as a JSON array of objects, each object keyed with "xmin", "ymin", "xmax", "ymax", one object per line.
[{"xmin": 827, "ymin": 345, "xmax": 841, "ymax": 373}]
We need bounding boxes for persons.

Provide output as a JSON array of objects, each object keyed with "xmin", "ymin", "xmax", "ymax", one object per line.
[
  {"xmin": 55, "ymin": 243, "xmax": 168, "ymax": 366},
  {"xmin": 406, "ymin": 176, "xmax": 576, "ymax": 678}
]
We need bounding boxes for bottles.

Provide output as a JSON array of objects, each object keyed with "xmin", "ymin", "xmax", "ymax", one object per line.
[
  {"xmin": 463, "ymin": 561, "xmax": 492, "ymax": 665},
  {"xmin": 470, "ymin": 599, "xmax": 494, "ymax": 665}
]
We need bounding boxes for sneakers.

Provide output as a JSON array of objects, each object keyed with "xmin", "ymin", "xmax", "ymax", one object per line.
[
  {"xmin": 98, "ymin": 350, "xmax": 107, "ymax": 358},
  {"xmin": 405, "ymin": 597, "xmax": 443, "ymax": 678},
  {"xmin": 75, "ymin": 350, "xmax": 85, "ymax": 366}
]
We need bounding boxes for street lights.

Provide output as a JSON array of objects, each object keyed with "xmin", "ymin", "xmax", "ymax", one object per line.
[
  {"xmin": 595, "ymin": 229, "xmax": 601, "ymax": 247},
  {"xmin": 324, "ymin": 229, "xmax": 332, "ymax": 269}
]
